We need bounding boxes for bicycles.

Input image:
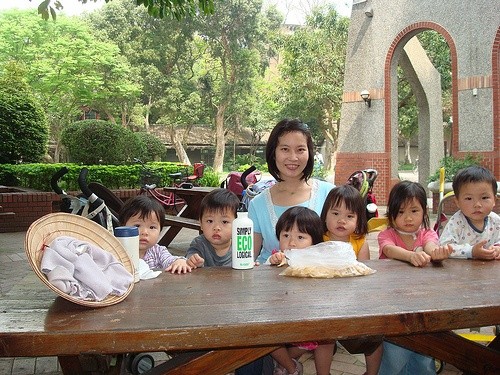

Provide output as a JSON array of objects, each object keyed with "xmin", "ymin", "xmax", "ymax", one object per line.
[{"xmin": 133, "ymin": 157, "xmax": 207, "ymax": 214}]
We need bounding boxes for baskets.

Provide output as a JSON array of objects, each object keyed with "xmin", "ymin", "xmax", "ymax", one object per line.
[{"xmin": 24, "ymin": 213, "xmax": 135, "ymax": 307}]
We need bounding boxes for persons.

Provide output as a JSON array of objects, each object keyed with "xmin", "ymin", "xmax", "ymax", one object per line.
[
  {"xmin": 262, "ymin": 205, "xmax": 324, "ymax": 375},
  {"xmin": 185, "ymin": 188, "xmax": 260, "ymax": 270},
  {"xmin": 118, "ymin": 195, "xmax": 192, "ymax": 275},
  {"xmin": 439, "ymin": 165, "xmax": 500, "ymax": 260},
  {"xmin": 378, "ymin": 180, "xmax": 454, "ymax": 375},
  {"xmin": 314, "ymin": 186, "xmax": 383, "ymax": 375},
  {"xmin": 248, "ymin": 118, "xmax": 370, "ymax": 266}
]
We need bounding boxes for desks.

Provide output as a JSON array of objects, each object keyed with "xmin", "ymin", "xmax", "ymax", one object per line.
[
  {"xmin": 157, "ymin": 187, "xmax": 219, "ymax": 246},
  {"xmin": 0, "ymin": 258, "xmax": 500, "ymax": 375}
]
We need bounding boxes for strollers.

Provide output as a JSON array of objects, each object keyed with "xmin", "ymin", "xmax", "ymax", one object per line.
[
  {"xmin": 50, "ymin": 166, "xmax": 129, "ymax": 232},
  {"xmin": 219, "ymin": 165, "xmax": 277, "ymax": 212}
]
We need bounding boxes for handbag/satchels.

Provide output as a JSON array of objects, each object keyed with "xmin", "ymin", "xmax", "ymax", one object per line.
[
  {"xmin": 226, "ymin": 172, "xmax": 261, "ymax": 195},
  {"xmin": 368, "ymin": 190, "xmax": 378, "ymax": 218}
]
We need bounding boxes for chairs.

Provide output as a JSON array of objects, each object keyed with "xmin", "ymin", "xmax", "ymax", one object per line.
[{"xmin": 184, "ymin": 163, "xmax": 206, "ymax": 181}]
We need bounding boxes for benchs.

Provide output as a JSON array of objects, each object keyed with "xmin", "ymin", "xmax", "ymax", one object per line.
[{"xmin": 165, "ymin": 215, "xmax": 202, "ymax": 231}]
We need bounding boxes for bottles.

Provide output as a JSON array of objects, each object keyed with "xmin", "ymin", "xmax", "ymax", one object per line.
[
  {"xmin": 114, "ymin": 227, "xmax": 147, "ymax": 276},
  {"xmin": 365, "ymin": 203, "xmax": 378, "ymax": 224},
  {"xmin": 231, "ymin": 202, "xmax": 255, "ymax": 270}
]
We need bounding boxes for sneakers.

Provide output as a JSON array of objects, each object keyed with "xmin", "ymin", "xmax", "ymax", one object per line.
[
  {"xmin": 274, "ymin": 368, "xmax": 288, "ymax": 375},
  {"xmin": 289, "ymin": 359, "xmax": 303, "ymax": 375}
]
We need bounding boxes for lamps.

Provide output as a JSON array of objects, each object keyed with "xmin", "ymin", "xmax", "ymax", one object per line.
[
  {"xmin": 361, "ymin": 90, "xmax": 371, "ymax": 107},
  {"xmin": 365, "ymin": 8, "xmax": 373, "ymax": 18}
]
